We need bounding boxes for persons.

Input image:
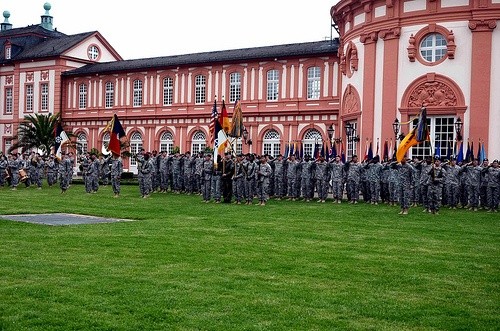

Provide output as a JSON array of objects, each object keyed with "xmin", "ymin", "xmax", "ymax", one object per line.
[
  {"xmin": 82, "ymin": 151, "xmax": 122, "ymax": 198},
  {"xmin": 0, "ymin": 151, "xmax": 75, "ymax": 194},
  {"xmin": 136, "ymin": 149, "xmax": 500, "ymax": 215}
]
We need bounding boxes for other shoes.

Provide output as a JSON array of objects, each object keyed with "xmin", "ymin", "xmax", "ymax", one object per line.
[
  {"xmin": 441, "ymin": 203, "xmax": 498, "ymax": 213},
  {"xmin": 213, "ymin": 200, "xmax": 216, "ymax": 203},
  {"xmin": 249, "ymin": 201, "xmax": 252, "ymax": 205},
  {"xmin": 237, "ymin": 202, "xmax": 241, "ymax": 204},
  {"xmin": 245, "ymin": 201, "xmax": 248, "ymax": 205},
  {"xmin": 261, "ymin": 201, "xmax": 265, "ymax": 206},
  {"xmin": 435, "ymin": 212, "xmax": 439, "ymax": 215},
  {"xmin": 255, "ymin": 201, "xmax": 261, "ymax": 205},
  {"xmin": 398, "ymin": 210, "xmax": 403, "ymax": 215},
  {"xmin": 202, "ymin": 200, "xmax": 205, "ymax": 203},
  {"xmin": 152, "ymin": 188, "xmax": 202, "ymax": 196},
  {"xmin": 216, "ymin": 200, "xmax": 220, "ymax": 204},
  {"xmin": 423, "ymin": 209, "xmax": 427, "ymax": 212},
  {"xmin": 206, "ymin": 200, "xmax": 209, "ymax": 203},
  {"xmin": 233, "ymin": 201, "xmax": 237, "ymax": 204},
  {"xmin": 275, "ymin": 195, "xmax": 398, "ymax": 206},
  {"xmin": 1, "ymin": 184, "xmax": 149, "ymax": 199},
  {"xmin": 403, "ymin": 210, "xmax": 408, "ymax": 215}
]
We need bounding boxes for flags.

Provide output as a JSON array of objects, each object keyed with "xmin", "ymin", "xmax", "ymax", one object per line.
[
  {"xmin": 54, "ymin": 120, "xmax": 71, "ymax": 160},
  {"xmin": 312, "ymin": 138, "xmax": 346, "ymax": 164},
  {"xmin": 364, "ymin": 138, "xmax": 397, "ymax": 161},
  {"xmin": 108, "ymin": 114, "xmax": 129, "ymax": 159},
  {"xmin": 434, "ymin": 139, "xmax": 486, "ymax": 165},
  {"xmin": 396, "ymin": 118, "xmax": 430, "ymax": 163},
  {"xmin": 208, "ymin": 94, "xmax": 246, "ymax": 169},
  {"xmin": 284, "ymin": 139, "xmax": 303, "ymax": 159}
]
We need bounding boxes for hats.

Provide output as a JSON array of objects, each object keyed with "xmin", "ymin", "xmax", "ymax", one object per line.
[{"xmin": 160, "ymin": 149, "xmax": 500, "ymax": 169}]
